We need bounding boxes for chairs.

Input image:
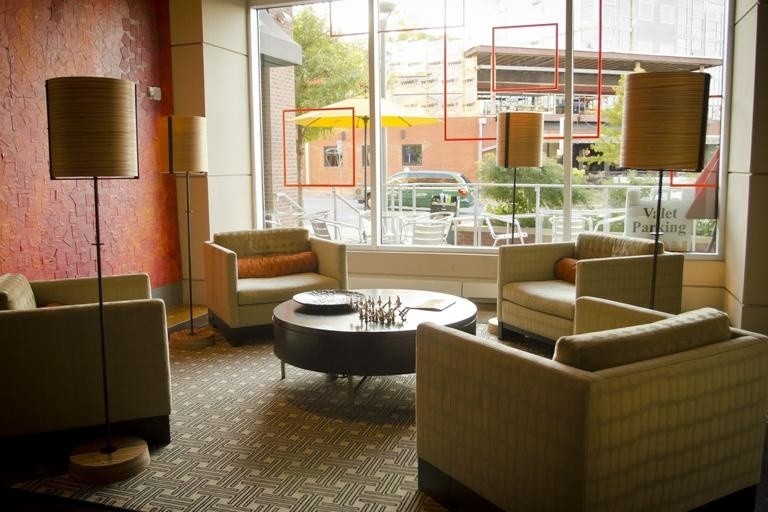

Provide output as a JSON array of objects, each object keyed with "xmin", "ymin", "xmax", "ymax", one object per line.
[
  {"xmin": 414, "ymin": 293, "xmax": 768, "ymax": 512},
  {"xmin": 0, "ymin": 271, "xmax": 173, "ymax": 445},
  {"xmin": 202, "ymin": 228, "xmax": 347, "ymax": 347}
]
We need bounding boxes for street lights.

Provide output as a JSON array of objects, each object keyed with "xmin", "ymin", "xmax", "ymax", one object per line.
[{"xmin": 374, "ymin": 0, "xmax": 395, "ymax": 227}]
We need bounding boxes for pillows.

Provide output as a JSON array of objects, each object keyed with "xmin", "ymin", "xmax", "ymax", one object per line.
[
  {"xmin": 237, "ymin": 250, "xmax": 318, "ymax": 279},
  {"xmin": 213, "ymin": 226, "xmax": 311, "ymax": 260},
  {"xmin": 553, "ymin": 256, "xmax": 582, "ymax": 284},
  {"xmin": 573, "ymin": 231, "xmax": 664, "ymax": 260},
  {"xmin": 551, "ymin": 305, "xmax": 731, "ymax": 373},
  {"xmin": 0, "ymin": 273, "xmax": 38, "ymax": 312},
  {"xmin": 44, "ymin": 302, "xmax": 63, "ymax": 308}
]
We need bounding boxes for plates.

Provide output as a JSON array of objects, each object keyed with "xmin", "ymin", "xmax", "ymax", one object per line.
[{"xmin": 292, "ymin": 289, "xmax": 367, "ymax": 311}]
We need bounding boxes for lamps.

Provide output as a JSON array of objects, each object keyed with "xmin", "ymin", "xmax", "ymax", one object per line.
[
  {"xmin": 619, "ymin": 69, "xmax": 713, "ymax": 310},
  {"xmin": 44, "ymin": 75, "xmax": 153, "ymax": 485},
  {"xmin": 155, "ymin": 114, "xmax": 217, "ymax": 352},
  {"xmin": 486, "ymin": 112, "xmax": 544, "ymax": 336}
]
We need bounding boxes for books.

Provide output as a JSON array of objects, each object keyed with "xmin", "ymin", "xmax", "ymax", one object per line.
[{"xmin": 404, "ymin": 299, "xmax": 455, "ymax": 311}]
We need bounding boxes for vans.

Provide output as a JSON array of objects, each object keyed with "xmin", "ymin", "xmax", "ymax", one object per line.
[{"xmin": 357, "ymin": 171, "xmax": 474, "ymax": 212}]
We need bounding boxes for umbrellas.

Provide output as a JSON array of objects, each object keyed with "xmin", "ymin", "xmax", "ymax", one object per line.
[{"xmin": 291, "ymin": 97, "xmax": 443, "ymax": 212}]
